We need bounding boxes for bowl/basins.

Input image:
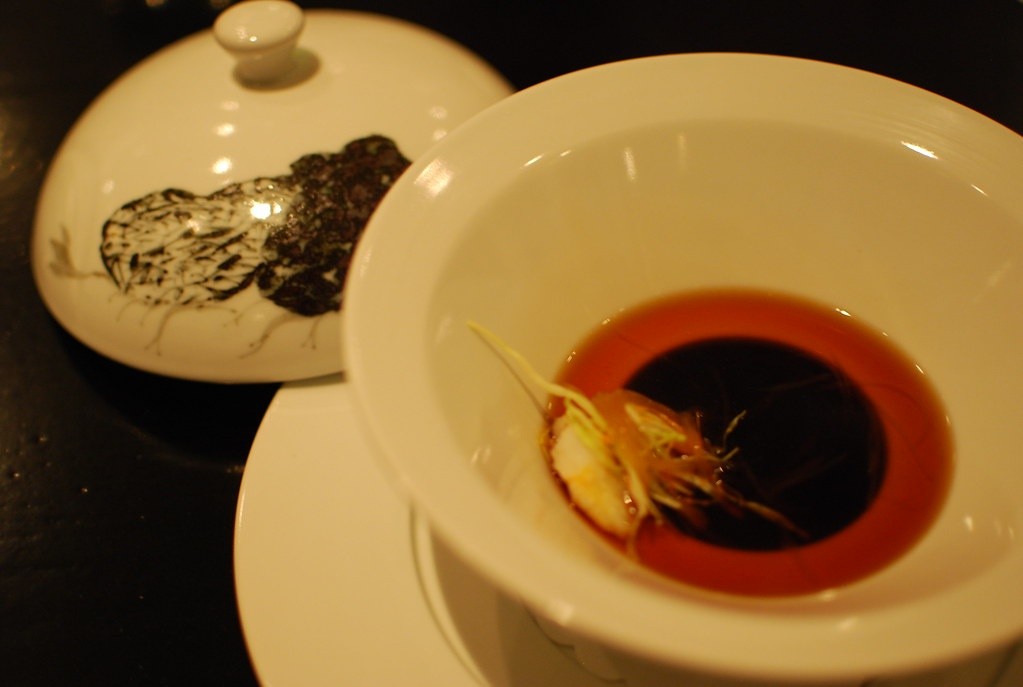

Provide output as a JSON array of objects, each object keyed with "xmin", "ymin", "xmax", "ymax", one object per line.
[{"xmin": 340, "ymin": 50, "xmax": 1023, "ymax": 687}]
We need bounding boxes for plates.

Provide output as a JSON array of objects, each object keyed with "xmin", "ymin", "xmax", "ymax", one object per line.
[{"xmin": 235, "ymin": 370, "xmax": 1023, "ymax": 687}]
